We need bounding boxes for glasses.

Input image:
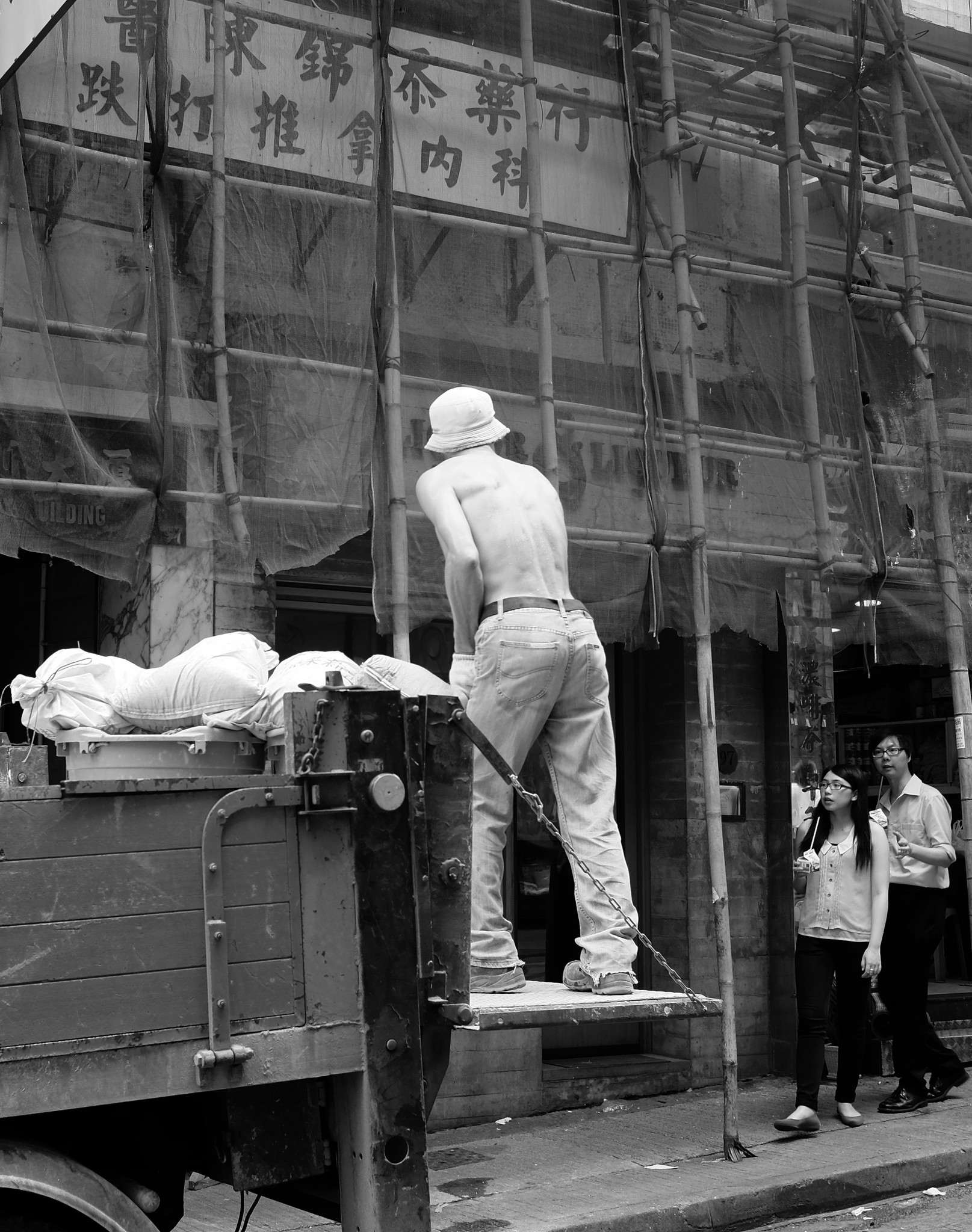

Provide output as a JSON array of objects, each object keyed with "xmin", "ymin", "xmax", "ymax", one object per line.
[
  {"xmin": 871, "ymin": 748, "xmax": 905, "ymax": 758},
  {"xmin": 817, "ymin": 781, "xmax": 855, "ymax": 790}
]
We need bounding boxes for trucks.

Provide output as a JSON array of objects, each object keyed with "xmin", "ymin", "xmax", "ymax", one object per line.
[{"xmin": 0, "ymin": 671, "xmax": 725, "ymax": 1232}]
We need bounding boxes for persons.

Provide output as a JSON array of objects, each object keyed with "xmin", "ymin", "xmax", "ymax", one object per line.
[
  {"xmin": 773, "ymin": 764, "xmax": 891, "ymax": 1130},
  {"xmin": 414, "ymin": 386, "xmax": 643, "ymax": 997},
  {"xmin": 869, "ymin": 729, "xmax": 970, "ymax": 1113}
]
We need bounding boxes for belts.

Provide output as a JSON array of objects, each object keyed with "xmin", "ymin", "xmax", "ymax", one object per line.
[{"xmin": 479, "ymin": 597, "xmax": 589, "ymax": 621}]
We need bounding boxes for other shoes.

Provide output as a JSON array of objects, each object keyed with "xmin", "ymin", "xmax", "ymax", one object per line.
[
  {"xmin": 773, "ymin": 1113, "xmax": 820, "ymax": 1132},
  {"xmin": 835, "ymin": 1104, "xmax": 864, "ymax": 1126}
]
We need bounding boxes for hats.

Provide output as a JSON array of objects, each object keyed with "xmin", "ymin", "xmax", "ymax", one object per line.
[{"xmin": 423, "ymin": 387, "xmax": 510, "ymax": 451}]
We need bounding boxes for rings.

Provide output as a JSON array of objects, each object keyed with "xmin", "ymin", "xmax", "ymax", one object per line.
[{"xmin": 870, "ymin": 969, "xmax": 874, "ymax": 971}]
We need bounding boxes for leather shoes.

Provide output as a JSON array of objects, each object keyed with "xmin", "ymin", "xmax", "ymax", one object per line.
[
  {"xmin": 877, "ymin": 1077, "xmax": 930, "ymax": 1113},
  {"xmin": 927, "ymin": 1045, "xmax": 969, "ymax": 1102}
]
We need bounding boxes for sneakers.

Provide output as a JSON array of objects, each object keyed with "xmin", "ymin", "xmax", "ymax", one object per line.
[
  {"xmin": 562, "ymin": 959, "xmax": 635, "ymax": 994},
  {"xmin": 470, "ymin": 965, "xmax": 525, "ymax": 993}
]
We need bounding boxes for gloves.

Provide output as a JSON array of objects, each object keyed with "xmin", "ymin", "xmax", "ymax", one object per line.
[{"xmin": 450, "ymin": 652, "xmax": 478, "ymax": 700}]
